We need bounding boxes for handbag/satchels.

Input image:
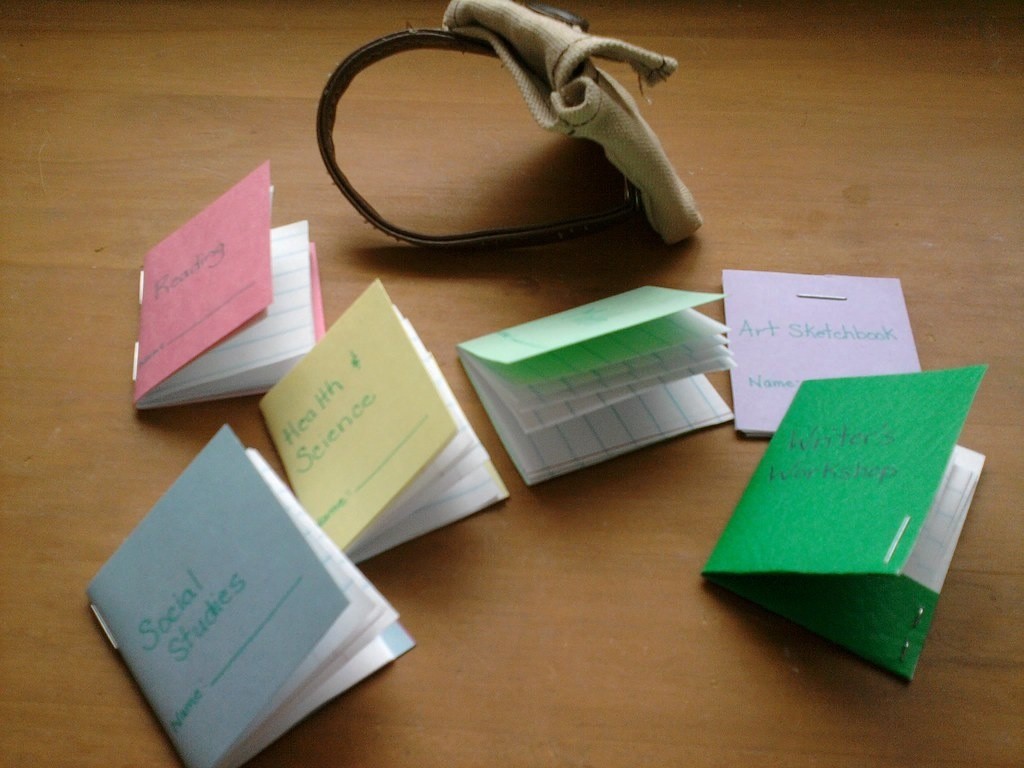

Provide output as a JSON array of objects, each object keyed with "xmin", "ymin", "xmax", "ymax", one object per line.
[{"xmin": 317, "ymin": 0, "xmax": 703, "ymax": 247}]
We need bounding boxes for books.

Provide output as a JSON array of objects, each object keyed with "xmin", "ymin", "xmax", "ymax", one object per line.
[
  {"xmin": 84, "ymin": 423, "xmax": 415, "ymax": 768},
  {"xmin": 454, "ymin": 284, "xmax": 738, "ymax": 486},
  {"xmin": 258, "ymin": 276, "xmax": 509, "ymax": 566},
  {"xmin": 722, "ymin": 269, "xmax": 921, "ymax": 440},
  {"xmin": 699, "ymin": 361, "xmax": 991, "ymax": 680},
  {"xmin": 131, "ymin": 157, "xmax": 329, "ymax": 413}
]
